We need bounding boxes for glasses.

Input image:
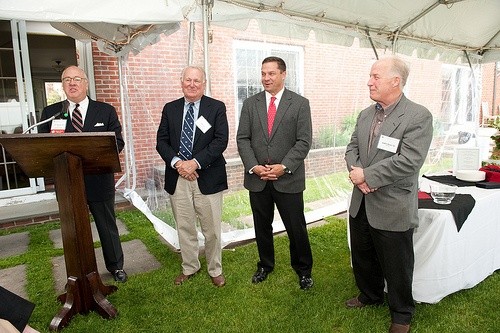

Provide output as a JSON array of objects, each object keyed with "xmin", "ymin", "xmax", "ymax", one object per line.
[{"xmin": 63, "ymin": 77, "xmax": 86, "ymax": 83}]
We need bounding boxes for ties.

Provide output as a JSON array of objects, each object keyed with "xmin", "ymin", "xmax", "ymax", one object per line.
[
  {"xmin": 72, "ymin": 104, "xmax": 83, "ymax": 133},
  {"xmin": 267, "ymin": 97, "xmax": 277, "ymax": 138},
  {"xmin": 178, "ymin": 102, "xmax": 194, "ymax": 161}
]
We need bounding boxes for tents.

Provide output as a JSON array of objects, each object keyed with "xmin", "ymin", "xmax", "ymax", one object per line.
[
  {"xmin": 187, "ymin": 0, "xmax": 500, "ymax": 95},
  {"xmin": 0, "ymin": 0, "xmax": 184, "ymax": 194}
]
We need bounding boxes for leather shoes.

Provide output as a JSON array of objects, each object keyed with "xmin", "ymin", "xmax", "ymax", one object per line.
[
  {"xmin": 299, "ymin": 276, "xmax": 313, "ymax": 290},
  {"xmin": 389, "ymin": 322, "xmax": 410, "ymax": 333},
  {"xmin": 211, "ymin": 275, "xmax": 225, "ymax": 287},
  {"xmin": 346, "ymin": 297, "xmax": 367, "ymax": 308},
  {"xmin": 111, "ymin": 269, "xmax": 127, "ymax": 284},
  {"xmin": 252, "ymin": 267, "xmax": 273, "ymax": 284},
  {"xmin": 174, "ymin": 268, "xmax": 201, "ymax": 285}
]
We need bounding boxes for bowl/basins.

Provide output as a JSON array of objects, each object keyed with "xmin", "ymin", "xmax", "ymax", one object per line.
[{"xmin": 455, "ymin": 170, "xmax": 485, "ymax": 182}]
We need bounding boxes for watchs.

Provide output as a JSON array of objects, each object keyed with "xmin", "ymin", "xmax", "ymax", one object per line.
[{"xmin": 282, "ymin": 163, "xmax": 290, "ymax": 174}]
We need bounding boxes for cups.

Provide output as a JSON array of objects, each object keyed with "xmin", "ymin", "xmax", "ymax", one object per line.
[
  {"xmin": 430, "ymin": 183, "xmax": 458, "ymax": 205},
  {"xmin": 453, "ymin": 148, "xmax": 481, "ymax": 177}
]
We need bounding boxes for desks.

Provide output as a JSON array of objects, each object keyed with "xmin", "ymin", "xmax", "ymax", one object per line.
[{"xmin": 348, "ymin": 169, "xmax": 500, "ymax": 305}]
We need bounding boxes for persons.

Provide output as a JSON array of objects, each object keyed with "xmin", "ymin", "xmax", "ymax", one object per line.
[
  {"xmin": 236, "ymin": 56, "xmax": 314, "ymax": 291},
  {"xmin": 38, "ymin": 65, "xmax": 128, "ymax": 281},
  {"xmin": 343, "ymin": 58, "xmax": 433, "ymax": 333},
  {"xmin": 155, "ymin": 67, "xmax": 229, "ymax": 287}
]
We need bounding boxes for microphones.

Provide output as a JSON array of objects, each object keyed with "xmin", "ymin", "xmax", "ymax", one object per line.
[{"xmin": 60, "ymin": 100, "xmax": 71, "ymax": 119}]
